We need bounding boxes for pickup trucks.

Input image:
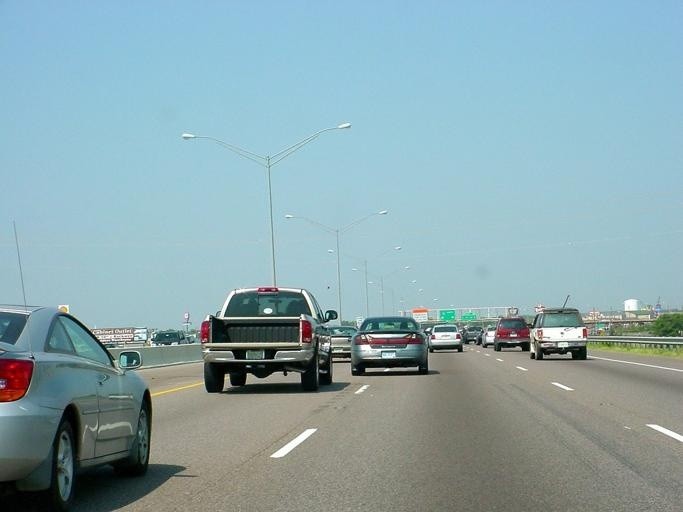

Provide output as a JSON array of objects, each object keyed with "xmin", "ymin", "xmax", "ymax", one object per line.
[{"xmin": 196, "ymin": 282, "xmax": 339, "ymax": 395}]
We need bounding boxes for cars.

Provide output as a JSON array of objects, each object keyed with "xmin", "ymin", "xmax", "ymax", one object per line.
[
  {"xmin": 457, "ymin": 323, "xmax": 499, "ymax": 348},
  {"xmin": 2, "ymin": 301, "xmax": 154, "ymax": 493},
  {"xmin": 426, "ymin": 323, "xmax": 467, "ymax": 356},
  {"xmin": 349, "ymin": 313, "xmax": 432, "ymax": 374},
  {"xmin": 143, "ymin": 327, "xmax": 192, "ymax": 352},
  {"xmin": 320, "ymin": 320, "xmax": 357, "ymax": 363},
  {"xmin": 528, "ymin": 306, "xmax": 592, "ymax": 362}
]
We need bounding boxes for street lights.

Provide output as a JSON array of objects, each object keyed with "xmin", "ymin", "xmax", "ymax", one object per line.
[
  {"xmin": 280, "ymin": 206, "xmax": 389, "ymax": 316},
  {"xmin": 323, "ymin": 241, "xmax": 459, "ymax": 314},
  {"xmin": 178, "ymin": 121, "xmax": 353, "ymax": 287}
]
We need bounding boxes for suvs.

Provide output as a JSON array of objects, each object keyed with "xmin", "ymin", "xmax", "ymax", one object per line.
[{"xmin": 491, "ymin": 314, "xmax": 533, "ymax": 354}]
